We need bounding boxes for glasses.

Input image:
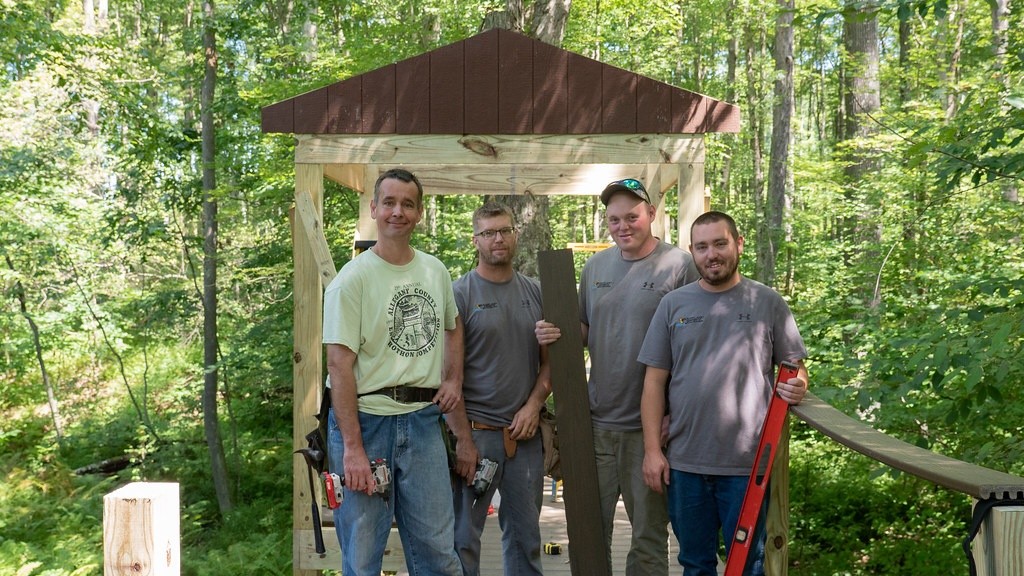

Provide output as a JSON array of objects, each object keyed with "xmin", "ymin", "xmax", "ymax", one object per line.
[
  {"xmin": 474, "ymin": 227, "xmax": 517, "ymax": 239},
  {"xmin": 607, "ymin": 179, "xmax": 652, "ymax": 204}
]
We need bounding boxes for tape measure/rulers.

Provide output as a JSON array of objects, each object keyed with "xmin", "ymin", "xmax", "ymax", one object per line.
[{"xmin": 543, "ymin": 541, "xmax": 563, "ymax": 555}]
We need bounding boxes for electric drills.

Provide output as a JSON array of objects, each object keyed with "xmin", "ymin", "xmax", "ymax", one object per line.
[
  {"xmin": 320, "ymin": 459, "xmax": 393, "ymax": 511},
  {"xmin": 449, "ymin": 450, "xmax": 498, "ymax": 509}
]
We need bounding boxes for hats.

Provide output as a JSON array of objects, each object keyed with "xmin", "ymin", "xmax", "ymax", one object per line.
[{"xmin": 600, "ymin": 185, "xmax": 650, "ymax": 205}]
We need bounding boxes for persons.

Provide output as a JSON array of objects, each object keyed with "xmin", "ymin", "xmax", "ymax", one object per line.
[
  {"xmin": 443, "ymin": 202, "xmax": 552, "ymax": 576},
  {"xmin": 321, "ymin": 169, "xmax": 462, "ymax": 576},
  {"xmin": 637, "ymin": 210, "xmax": 809, "ymax": 576},
  {"xmin": 535, "ymin": 179, "xmax": 702, "ymax": 576}
]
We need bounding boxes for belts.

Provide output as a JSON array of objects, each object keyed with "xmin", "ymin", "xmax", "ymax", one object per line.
[
  {"xmin": 356, "ymin": 386, "xmax": 441, "ymax": 402},
  {"xmin": 470, "ymin": 422, "xmax": 497, "ymax": 431}
]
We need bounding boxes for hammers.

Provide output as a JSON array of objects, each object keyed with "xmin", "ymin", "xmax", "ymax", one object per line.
[{"xmin": 291, "ymin": 448, "xmax": 327, "ymax": 554}]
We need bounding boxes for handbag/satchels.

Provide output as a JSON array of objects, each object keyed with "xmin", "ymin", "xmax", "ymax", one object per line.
[{"xmin": 539, "ymin": 405, "xmax": 559, "ymax": 475}]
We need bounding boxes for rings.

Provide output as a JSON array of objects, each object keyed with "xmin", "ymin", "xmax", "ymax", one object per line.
[{"xmin": 528, "ymin": 432, "xmax": 532, "ymax": 434}]
